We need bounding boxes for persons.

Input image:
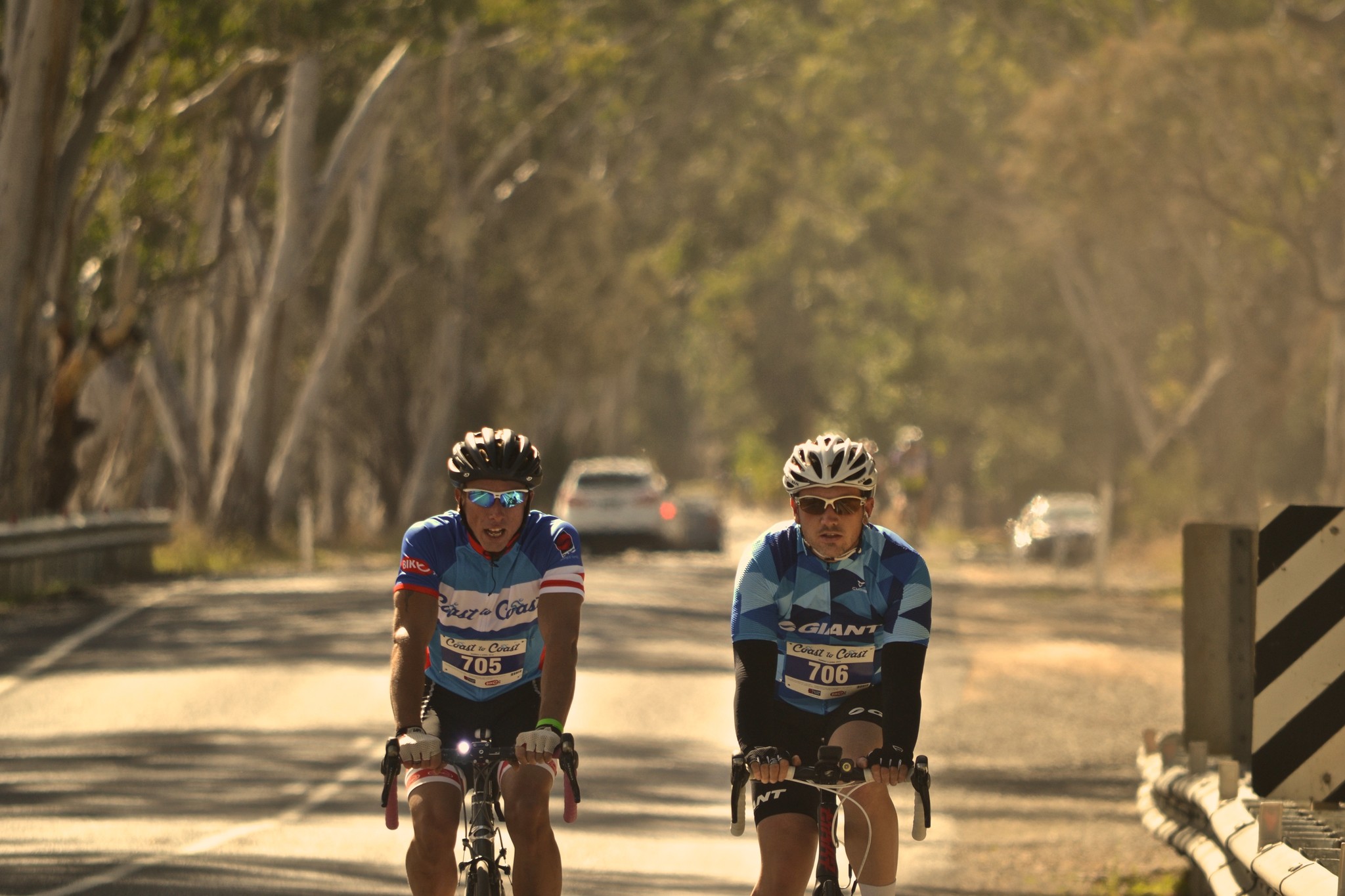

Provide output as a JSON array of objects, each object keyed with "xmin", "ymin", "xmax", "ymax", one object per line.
[
  {"xmin": 389, "ymin": 427, "xmax": 585, "ymax": 896},
  {"xmin": 730, "ymin": 436, "xmax": 932, "ymax": 896}
]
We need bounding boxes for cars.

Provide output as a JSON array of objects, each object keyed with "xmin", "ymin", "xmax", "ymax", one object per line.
[
  {"xmin": 1005, "ymin": 490, "xmax": 1104, "ymax": 565},
  {"xmin": 673, "ymin": 488, "xmax": 724, "ymax": 555},
  {"xmin": 550, "ymin": 454, "xmax": 675, "ymax": 555}
]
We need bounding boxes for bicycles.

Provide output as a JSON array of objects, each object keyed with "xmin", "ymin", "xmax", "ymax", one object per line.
[
  {"xmin": 379, "ymin": 731, "xmax": 582, "ymax": 896},
  {"xmin": 728, "ymin": 743, "xmax": 932, "ymax": 896}
]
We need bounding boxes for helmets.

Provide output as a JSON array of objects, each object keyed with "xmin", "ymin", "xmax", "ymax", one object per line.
[
  {"xmin": 783, "ymin": 435, "xmax": 877, "ymax": 499},
  {"xmin": 447, "ymin": 427, "xmax": 543, "ymax": 493}
]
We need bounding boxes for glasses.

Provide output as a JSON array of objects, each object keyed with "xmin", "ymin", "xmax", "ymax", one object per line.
[
  {"xmin": 791, "ymin": 495, "xmax": 867, "ymax": 516},
  {"xmin": 462, "ymin": 488, "xmax": 529, "ymax": 508}
]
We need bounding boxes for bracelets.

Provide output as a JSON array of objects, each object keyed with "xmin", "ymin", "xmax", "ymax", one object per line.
[{"xmin": 537, "ymin": 718, "xmax": 564, "ymax": 734}]
